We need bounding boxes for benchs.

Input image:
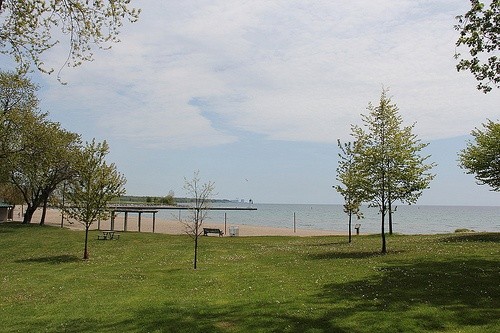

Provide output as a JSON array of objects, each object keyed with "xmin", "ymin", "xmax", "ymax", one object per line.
[
  {"xmin": 113, "ymin": 234, "xmax": 120, "ymax": 239},
  {"xmin": 203, "ymin": 228, "xmax": 224, "ymax": 238},
  {"xmin": 98, "ymin": 235, "xmax": 107, "ymax": 240}
]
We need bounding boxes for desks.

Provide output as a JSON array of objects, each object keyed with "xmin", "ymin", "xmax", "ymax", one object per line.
[{"xmin": 103, "ymin": 232, "xmax": 115, "ymax": 239}]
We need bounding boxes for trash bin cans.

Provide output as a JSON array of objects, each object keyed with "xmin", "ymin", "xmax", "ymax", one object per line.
[{"xmin": 229, "ymin": 226, "xmax": 240, "ymax": 236}]
